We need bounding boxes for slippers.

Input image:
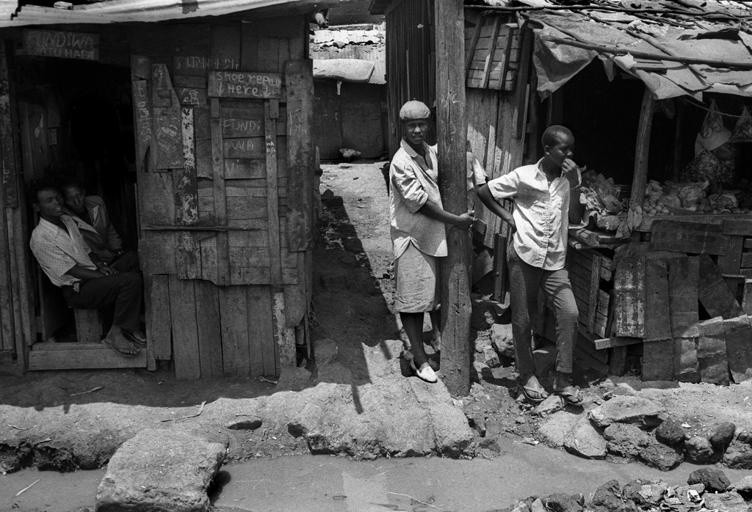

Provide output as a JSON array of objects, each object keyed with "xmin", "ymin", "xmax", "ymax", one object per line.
[
  {"xmin": 122, "ymin": 330, "xmax": 146, "ymax": 345},
  {"xmin": 104, "ymin": 337, "xmax": 141, "ymax": 358},
  {"xmin": 553, "ymin": 384, "xmax": 585, "ymax": 406},
  {"xmin": 516, "ymin": 376, "xmax": 548, "ymax": 401}
]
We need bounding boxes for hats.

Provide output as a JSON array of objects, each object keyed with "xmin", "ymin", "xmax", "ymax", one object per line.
[{"xmin": 399, "ymin": 100, "xmax": 432, "ymax": 121}]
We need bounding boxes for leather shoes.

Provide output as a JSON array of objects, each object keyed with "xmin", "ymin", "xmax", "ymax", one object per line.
[
  {"xmin": 405, "ymin": 351, "xmax": 440, "ymax": 370},
  {"xmin": 409, "ymin": 357, "xmax": 437, "ymax": 382},
  {"xmin": 428, "ymin": 329, "xmax": 441, "ymax": 354}
]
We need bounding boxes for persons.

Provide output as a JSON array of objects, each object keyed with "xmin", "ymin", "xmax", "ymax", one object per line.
[
  {"xmin": 29, "ymin": 183, "xmax": 146, "ymax": 358},
  {"xmin": 477, "ymin": 125, "xmax": 587, "ymax": 407},
  {"xmin": 388, "ymin": 100, "xmax": 478, "ymax": 383},
  {"xmin": 59, "ymin": 176, "xmax": 145, "ymax": 330},
  {"xmin": 465, "ymin": 140, "xmax": 486, "ymax": 210}
]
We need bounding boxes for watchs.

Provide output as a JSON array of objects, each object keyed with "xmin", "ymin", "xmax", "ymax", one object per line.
[{"xmin": 570, "ymin": 184, "xmax": 581, "ymax": 192}]
